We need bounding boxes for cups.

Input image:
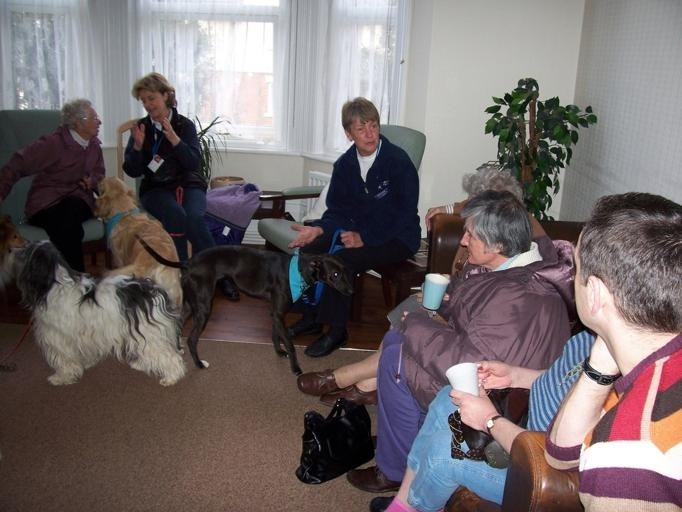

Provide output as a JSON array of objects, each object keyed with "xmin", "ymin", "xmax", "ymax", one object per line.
[
  {"xmin": 419, "ymin": 271, "xmax": 450, "ymax": 312},
  {"xmin": 446, "ymin": 361, "xmax": 485, "ymax": 401}
]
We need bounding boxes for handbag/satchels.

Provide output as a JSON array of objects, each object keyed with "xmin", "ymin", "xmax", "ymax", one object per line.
[
  {"xmin": 448, "ymin": 389, "xmax": 528, "ymax": 461},
  {"xmin": 296, "ymin": 399, "xmax": 374, "ymax": 484}
]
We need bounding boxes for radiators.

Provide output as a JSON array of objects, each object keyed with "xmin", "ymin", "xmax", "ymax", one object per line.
[{"xmin": 307, "ymin": 171, "xmax": 332, "ymax": 214}]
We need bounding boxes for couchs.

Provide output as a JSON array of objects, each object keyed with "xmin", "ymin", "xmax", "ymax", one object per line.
[{"xmin": 428, "ymin": 211, "xmax": 612, "ymax": 512}]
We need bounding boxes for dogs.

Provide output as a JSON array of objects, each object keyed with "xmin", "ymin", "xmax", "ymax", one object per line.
[
  {"xmin": 133, "ymin": 233, "xmax": 356, "ymax": 375},
  {"xmin": 0, "ymin": 212, "xmax": 30, "ymax": 292},
  {"xmin": 90, "ymin": 176, "xmax": 186, "ymax": 320},
  {"xmin": 7, "ymin": 237, "xmax": 188, "ymax": 388}
]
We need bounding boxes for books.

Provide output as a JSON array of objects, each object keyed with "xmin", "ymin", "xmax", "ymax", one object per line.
[{"xmin": 240, "ymin": 218, "xmax": 267, "ymax": 246}]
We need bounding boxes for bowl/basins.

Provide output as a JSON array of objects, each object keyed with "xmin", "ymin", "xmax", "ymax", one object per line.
[{"xmin": 210, "ymin": 176, "xmax": 244, "ymax": 190}]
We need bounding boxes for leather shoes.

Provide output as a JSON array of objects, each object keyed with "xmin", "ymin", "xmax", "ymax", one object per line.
[
  {"xmin": 347, "ymin": 465, "xmax": 401, "ymax": 492},
  {"xmin": 221, "ymin": 277, "xmax": 240, "ymax": 300},
  {"xmin": 305, "ymin": 330, "xmax": 348, "ymax": 357},
  {"xmin": 297, "ymin": 369, "xmax": 378, "ymax": 406},
  {"xmin": 285, "ymin": 314, "xmax": 324, "ymax": 339}
]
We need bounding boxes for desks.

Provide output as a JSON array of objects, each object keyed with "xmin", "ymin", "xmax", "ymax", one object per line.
[{"xmin": 249, "ymin": 189, "xmax": 285, "ymax": 254}]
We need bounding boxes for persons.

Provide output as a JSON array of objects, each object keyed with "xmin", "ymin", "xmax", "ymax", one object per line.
[
  {"xmin": 1, "ymin": 98, "xmax": 107, "ymax": 276},
  {"xmin": 297, "ymin": 166, "xmax": 682, "ymax": 511},
  {"xmin": 285, "ymin": 97, "xmax": 422, "ymax": 357},
  {"xmin": 119, "ymin": 72, "xmax": 241, "ymax": 308}
]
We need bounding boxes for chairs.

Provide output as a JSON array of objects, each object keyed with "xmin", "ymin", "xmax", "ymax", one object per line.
[
  {"xmin": 0, "ymin": 108, "xmax": 108, "ymax": 317},
  {"xmin": 257, "ymin": 125, "xmax": 429, "ymax": 323},
  {"xmin": 116, "ymin": 118, "xmax": 147, "ymax": 205}
]
